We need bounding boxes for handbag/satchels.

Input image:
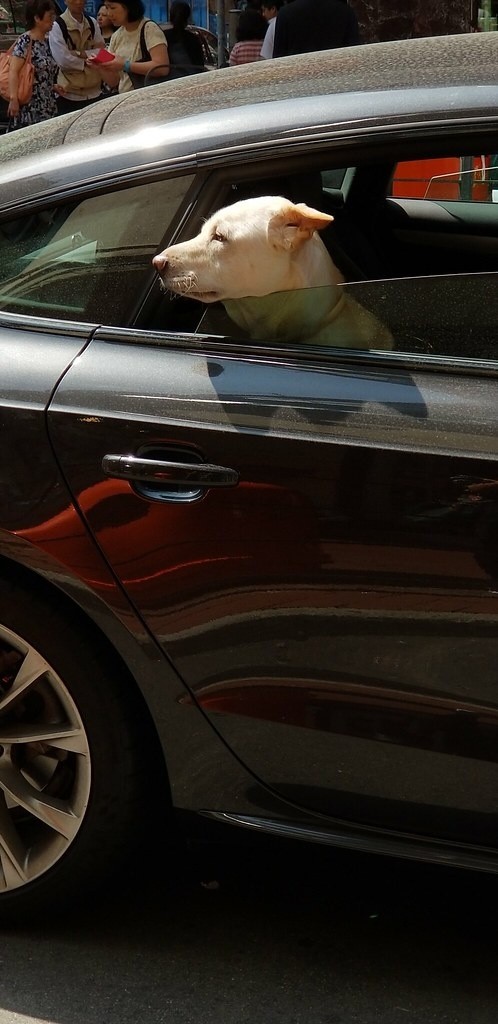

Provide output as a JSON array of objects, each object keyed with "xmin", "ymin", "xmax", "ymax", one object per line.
[
  {"xmin": 0, "ymin": 33, "xmax": 35, "ymax": 105},
  {"xmin": 123, "ymin": 20, "xmax": 174, "ymax": 89}
]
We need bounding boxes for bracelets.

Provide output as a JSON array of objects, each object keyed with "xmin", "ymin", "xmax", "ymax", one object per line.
[{"xmin": 124, "ymin": 59, "xmax": 130, "ymax": 73}]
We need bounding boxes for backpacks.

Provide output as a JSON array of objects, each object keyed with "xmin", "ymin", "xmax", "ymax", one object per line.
[{"xmin": 47, "ymin": 14, "xmax": 95, "ymax": 84}]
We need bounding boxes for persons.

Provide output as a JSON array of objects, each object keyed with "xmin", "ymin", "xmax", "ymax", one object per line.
[{"xmin": 5, "ymin": 0, "xmax": 359, "ymax": 132}]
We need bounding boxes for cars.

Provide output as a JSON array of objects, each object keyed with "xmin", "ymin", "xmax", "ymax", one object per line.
[{"xmin": 0, "ymin": 31, "xmax": 498, "ymax": 927}]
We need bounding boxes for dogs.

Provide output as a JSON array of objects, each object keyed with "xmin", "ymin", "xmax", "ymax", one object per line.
[{"xmin": 153, "ymin": 195, "xmax": 400, "ymax": 355}]
